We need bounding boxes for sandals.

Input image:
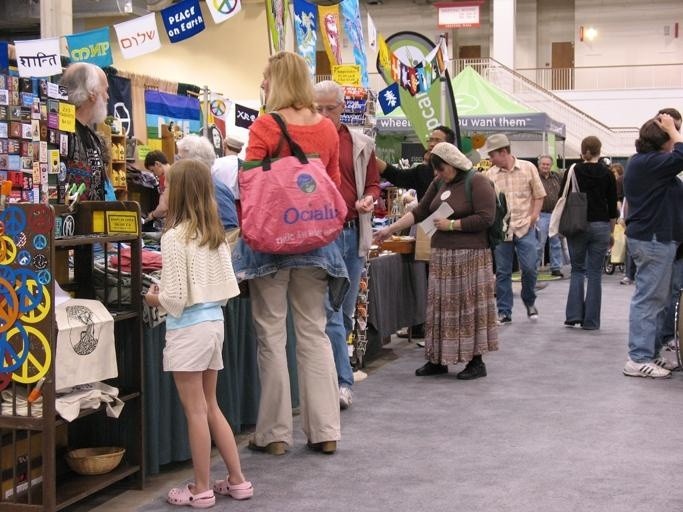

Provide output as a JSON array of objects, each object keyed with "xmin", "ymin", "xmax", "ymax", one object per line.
[
  {"xmin": 213, "ymin": 474, "xmax": 254, "ymax": 499},
  {"xmin": 167, "ymin": 483, "xmax": 216, "ymax": 509}
]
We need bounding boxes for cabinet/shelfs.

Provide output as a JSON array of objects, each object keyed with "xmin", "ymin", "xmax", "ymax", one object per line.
[
  {"xmin": 1, "ymin": 199, "xmax": 146, "ymax": 512},
  {"xmin": 70, "ymin": 219, "xmax": 431, "ymax": 473},
  {"xmin": 95, "ymin": 117, "xmax": 128, "ymax": 201}
]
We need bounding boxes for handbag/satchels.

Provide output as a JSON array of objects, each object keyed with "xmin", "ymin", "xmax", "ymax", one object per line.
[
  {"xmin": 548, "ymin": 197, "xmax": 566, "ymax": 238},
  {"xmin": 559, "ymin": 190, "xmax": 588, "ymax": 238},
  {"xmin": 610, "ymin": 223, "xmax": 627, "ymax": 265},
  {"xmin": 2, "ymin": 381, "xmax": 124, "ymax": 421}
]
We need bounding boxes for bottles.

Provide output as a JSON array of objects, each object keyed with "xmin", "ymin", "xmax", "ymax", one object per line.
[{"xmin": 341, "ymin": 114, "xmax": 363, "ymax": 124}]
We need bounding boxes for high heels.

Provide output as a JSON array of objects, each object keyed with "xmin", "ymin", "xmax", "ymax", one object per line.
[
  {"xmin": 307, "ymin": 440, "xmax": 336, "ymax": 453},
  {"xmin": 248, "ymin": 439, "xmax": 286, "ymax": 456}
]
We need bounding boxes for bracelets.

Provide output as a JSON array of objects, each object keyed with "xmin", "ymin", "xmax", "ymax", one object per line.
[{"xmin": 448, "ymin": 219, "xmax": 455, "ymax": 232}]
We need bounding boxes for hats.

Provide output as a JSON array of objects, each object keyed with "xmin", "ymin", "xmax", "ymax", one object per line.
[
  {"xmin": 431, "ymin": 142, "xmax": 473, "ymax": 173},
  {"xmin": 225, "ymin": 135, "xmax": 244, "ymax": 150},
  {"xmin": 480, "ymin": 134, "xmax": 509, "ymax": 153}
]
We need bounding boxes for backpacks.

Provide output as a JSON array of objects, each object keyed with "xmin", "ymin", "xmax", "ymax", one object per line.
[
  {"xmin": 237, "ymin": 153, "xmax": 348, "ymax": 254},
  {"xmin": 436, "ymin": 169, "xmax": 511, "ymax": 246}
]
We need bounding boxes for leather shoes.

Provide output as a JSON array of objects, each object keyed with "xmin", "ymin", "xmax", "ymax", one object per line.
[
  {"xmin": 415, "ymin": 362, "xmax": 449, "ymax": 376},
  {"xmin": 457, "ymin": 361, "xmax": 487, "ymax": 380}
]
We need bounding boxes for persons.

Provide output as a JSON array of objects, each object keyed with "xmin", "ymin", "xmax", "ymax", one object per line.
[
  {"xmin": 142, "ymin": 136, "xmax": 246, "ymax": 238},
  {"xmin": 373, "ymin": 126, "xmax": 455, "ymax": 348},
  {"xmin": 312, "ymin": 80, "xmax": 380, "ymax": 409},
  {"xmin": 533, "ymin": 155, "xmax": 638, "ymax": 285},
  {"xmin": 371, "ymin": 142, "xmax": 499, "ymax": 380},
  {"xmin": 144, "ymin": 157, "xmax": 254, "ymax": 509},
  {"xmin": 485, "ymin": 132, "xmax": 546, "ymax": 327},
  {"xmin": 56, "ymin": 62, "xmax": 144, "ymax": 257},
  {"xmin": 246, "ymin": 51, "xmax": 341, "ymax": 455},
  {"xmin": 622, "ymin": 107, "xmax": 683, "ymax": 379},
  {"xmin": 557, "ymin": 136, "xmax": 617, "ymax": 330}
]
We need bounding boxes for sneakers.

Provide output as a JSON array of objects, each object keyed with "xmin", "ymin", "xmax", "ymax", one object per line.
[
  {"xmin": 339, "ymin": 385, "xmax": 354, "ymax": 409},
  {"xmin": 527, "ymin": 306, "xmax": 539, "ymax": 320},
  {"xmin": 623, "ymin": 359, "xmax": 673, "ymax": 379},
  {"xmin": 652, "ymin": 357, "xmax": 680, "ymax": 371},
  {"xmin": 496, "ymin": 315, "xmax": 512, "ymax": 326}
]
